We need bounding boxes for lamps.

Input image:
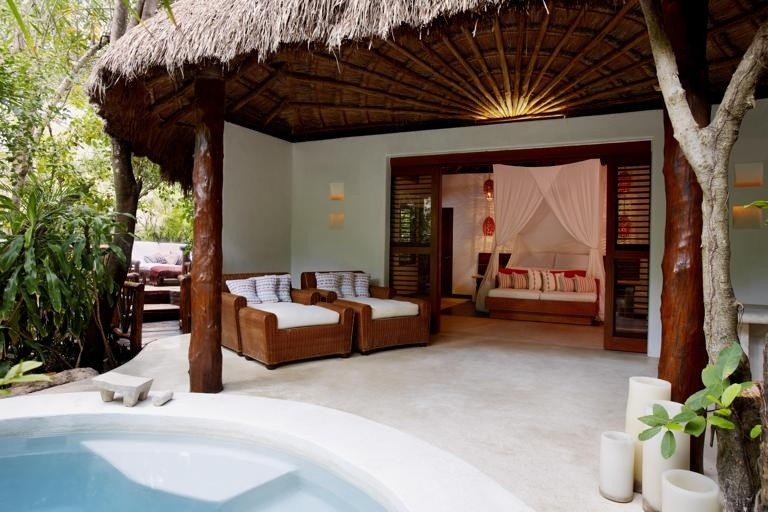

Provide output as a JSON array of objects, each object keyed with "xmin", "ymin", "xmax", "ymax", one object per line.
[
  {"xmin": 618, "ymin": 166, "xmax": 632, "ymax": 243},
  {"xmin": 482, "ymin": 172, "xmax": 496, "ymax": 237},
  {"xmin": 732, "ymin": 163, "xmax": 763, "ymax": 228},
  {"xmin": 328, "ymin": 183, "xmax": 346, "ymax": 231}
]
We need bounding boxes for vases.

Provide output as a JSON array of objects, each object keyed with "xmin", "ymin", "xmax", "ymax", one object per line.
[{"xmin": 599, "ymin": 374, "xmax": 721, "ymax": 512}]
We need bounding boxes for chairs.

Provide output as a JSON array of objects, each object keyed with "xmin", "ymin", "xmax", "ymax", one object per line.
[
  {"xmin": 300, "ymin": 271, "xmax": 431, "ymax": 355},
  {"xmin": 221, "ymin": 270, "xmax": 355, "ymax": 370},
  {"xmin": 127, "ymin": 242, "xmax": 191, "ymax": 286}
]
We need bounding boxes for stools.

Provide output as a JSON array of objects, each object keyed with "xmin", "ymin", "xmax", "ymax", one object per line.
[{"xmin": 91, "ymin": 371, "xmax": 154, "ymax": 407}]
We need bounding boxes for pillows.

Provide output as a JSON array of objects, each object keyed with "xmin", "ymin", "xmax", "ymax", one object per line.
[
  {"xmin": 496, "ymin": 269, "xmax": 597, "ymax": 294},
  {"xmin": 225, "ymin": 273, "xmax": 292, "ymax": 305},
  {"xmin": 314, "ymin": 272, "xmax": 370, "ymax": 298}
]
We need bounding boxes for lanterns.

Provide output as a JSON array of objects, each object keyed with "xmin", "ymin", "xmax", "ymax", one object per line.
[
  {"xmin": 619, "ymin": 212, "xmax": 632, "ymax": 244},
  {"xmin": 619, "ymin": 169, "xmax": 633, "ymax": 195},
  {"xmin": 482, "ymin": 214, "xmax": 496, "ymax": 240},
  {"xmin": 483, "ymin": 178, "xmax": 495, "ymax": 201}
]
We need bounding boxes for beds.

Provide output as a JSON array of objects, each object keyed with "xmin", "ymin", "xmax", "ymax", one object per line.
[{"xmin": 485, "ymin": 250, "xmax": 601, "ymax": 326}]
